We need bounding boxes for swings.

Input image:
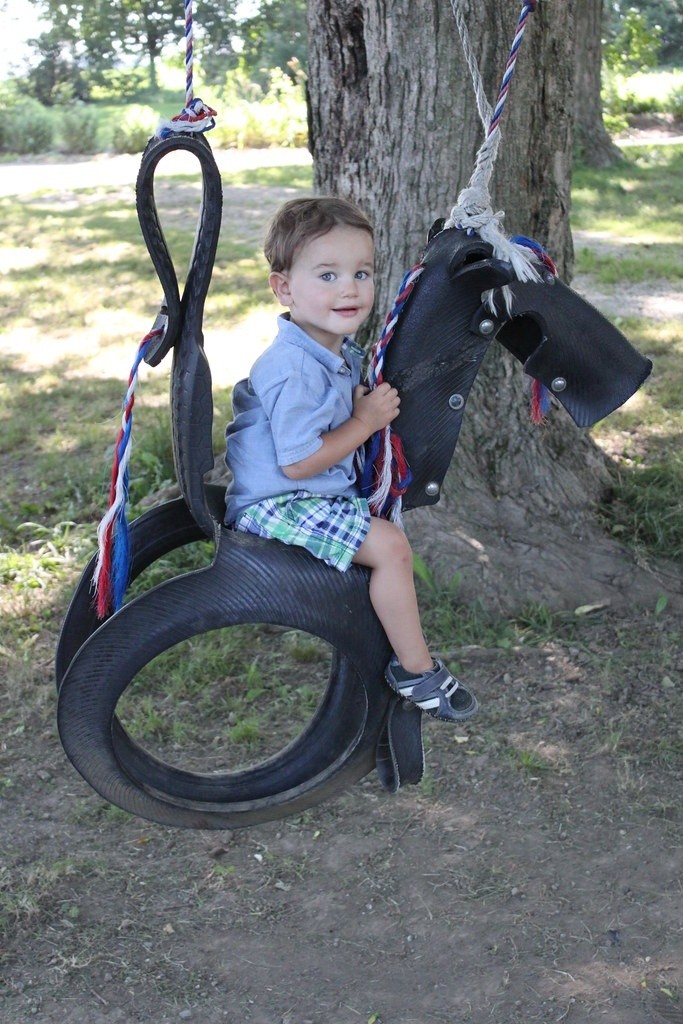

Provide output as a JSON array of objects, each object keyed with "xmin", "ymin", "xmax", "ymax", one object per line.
[{"xmin": 57, "ymin": 0, "xmax": 655, "ymax": 831}]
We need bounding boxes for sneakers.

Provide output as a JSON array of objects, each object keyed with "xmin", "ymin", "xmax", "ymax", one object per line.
[{"xmin": 384, "ymin": 653, "xmax": 479, "ymax": 722}]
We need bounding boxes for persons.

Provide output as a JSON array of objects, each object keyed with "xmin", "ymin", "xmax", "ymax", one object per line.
[{"xmin": 225, "ymin": 199, "xmax": 480, "ymax": 720}]
